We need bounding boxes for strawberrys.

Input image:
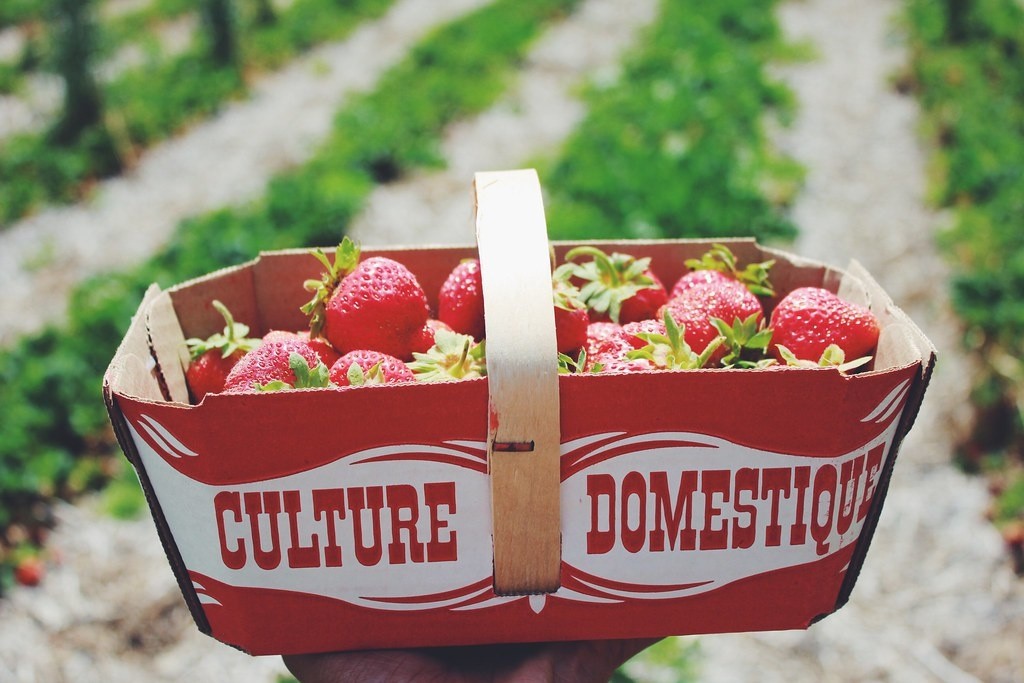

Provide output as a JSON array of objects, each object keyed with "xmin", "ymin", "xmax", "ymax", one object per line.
[{"xmin": 186, "ymin": 236, "xmax": 880, "ymax": 405}]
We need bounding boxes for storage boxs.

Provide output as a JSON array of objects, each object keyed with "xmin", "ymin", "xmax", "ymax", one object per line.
[{"xmin": 97, "ymin": 167, "xmax": 938, "ymax": 656}]
